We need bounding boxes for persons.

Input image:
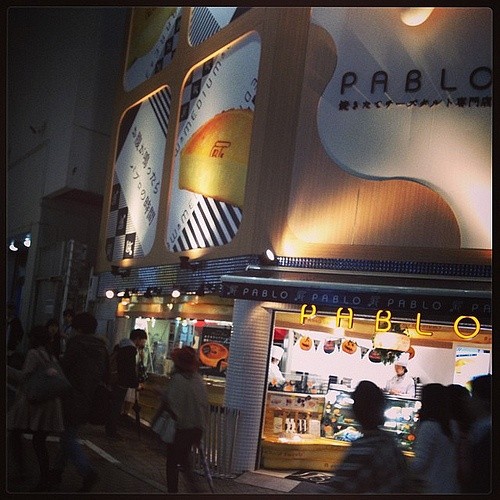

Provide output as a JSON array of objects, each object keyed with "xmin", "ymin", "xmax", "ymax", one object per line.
[
  {"xmin": 321, "ymin": 380, "xmax": 414, "ymax": 494},
  {"xmin": 7, "ymin": 303, "xmax": 110, "ymax": 493},
  {"xmin": 111, "ymin": 329, "xmax": 148, "ymax": 430},
  {"xmin": 162, "ymin": 345, "xmax": 209, "ymax": 493},
  {"xmin": 404, "ymin": 375, "xmax": 493, "ymax": 492}
]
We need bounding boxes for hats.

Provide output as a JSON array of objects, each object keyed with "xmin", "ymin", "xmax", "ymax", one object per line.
[{"xmin": 173, "ymin": 345, "xmax": 200, "ymax": 371}]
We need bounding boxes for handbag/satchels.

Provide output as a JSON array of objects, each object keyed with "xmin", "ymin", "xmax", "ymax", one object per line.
[{"xmin": 24, "ymin": 349, "xmax": 74, "ymax": 405}]
